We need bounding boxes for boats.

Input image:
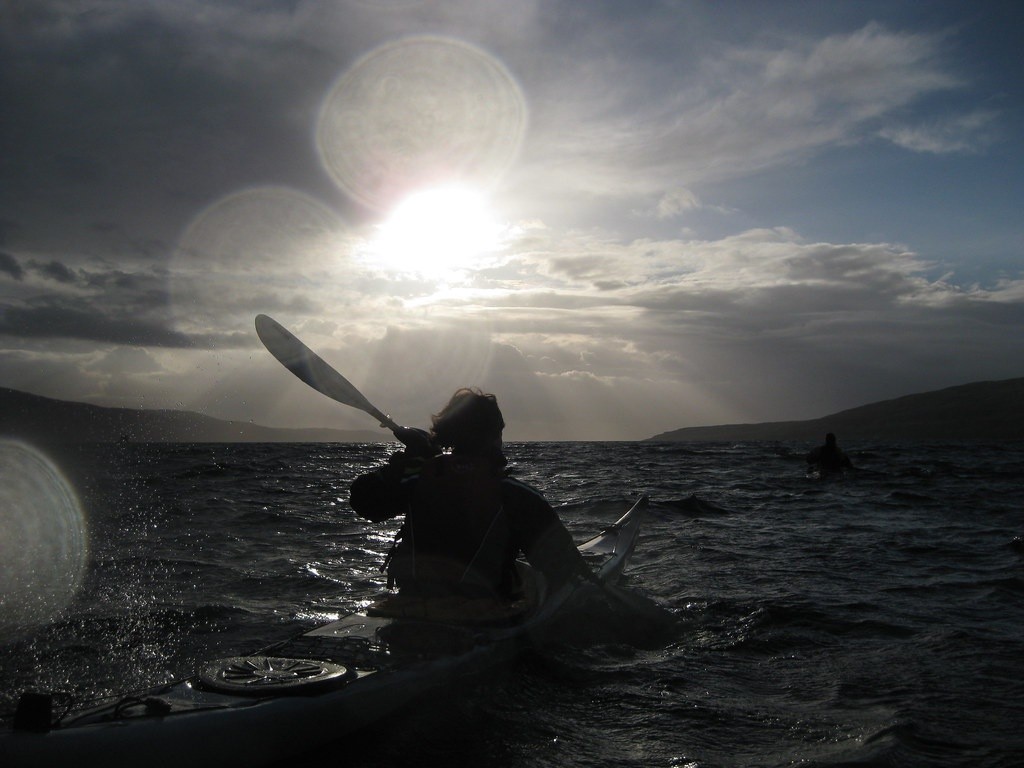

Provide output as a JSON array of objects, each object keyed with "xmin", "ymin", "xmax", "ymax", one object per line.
[{"xmin": 0, "ymin": 490, "xmax": 650, "ymax": 768}]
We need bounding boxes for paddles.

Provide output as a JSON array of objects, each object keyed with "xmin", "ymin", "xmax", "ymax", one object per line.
[{"xmin": 255, "ymin": 314, "xmax": 662, "ymax": 625}]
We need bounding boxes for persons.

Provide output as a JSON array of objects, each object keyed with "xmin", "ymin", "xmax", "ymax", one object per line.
[
  {"xmin": 350, "ymin": 390, "xmax": 589, "ymax": 612},
  {"xmin": 807, "ymin": 432, "xmax": 852, "ymax": 472}
]
